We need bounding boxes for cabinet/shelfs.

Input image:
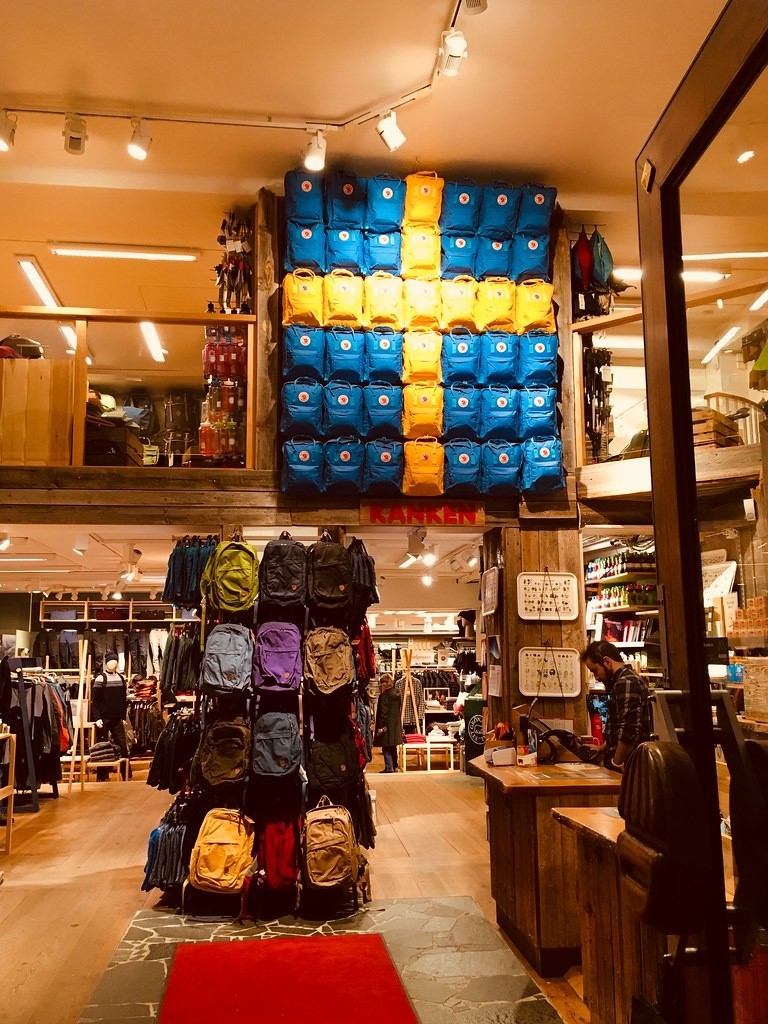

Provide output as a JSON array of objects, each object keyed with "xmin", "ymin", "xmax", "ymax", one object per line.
[
  {"xmin": 39, "ymin": 601, "xmax": 199, "ymax": 623},
  {"xmin": 584, "ymin": 570, "xmax": 658, "ymax": 691}
]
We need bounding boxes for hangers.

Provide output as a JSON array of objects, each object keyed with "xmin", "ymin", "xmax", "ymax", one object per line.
[
  {"xmin": 127, "ymin": 699, "xmax": 160, "ymax": 711},
  {"xmin": 10, "ymin": 670, "xmax": 62, "ymax": 685},
  {"xmin": 395, "ymin": 647, "xmax": 477, "ymax": 678}
]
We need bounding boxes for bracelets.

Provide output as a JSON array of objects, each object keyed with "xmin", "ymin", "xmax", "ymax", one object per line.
[{"xmin": 612, "ymin": 758, "xmax": 625, "ymax": 767}]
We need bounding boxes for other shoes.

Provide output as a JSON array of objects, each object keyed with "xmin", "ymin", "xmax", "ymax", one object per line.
[{"xmin": 380, "ymin": 769, "xmax": 393, "ymax": 773}]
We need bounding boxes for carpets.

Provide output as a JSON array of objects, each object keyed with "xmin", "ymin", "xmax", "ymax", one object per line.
[{"xmin": 153, "ymin": 933, "xmax": 421, "ymax": 1024}]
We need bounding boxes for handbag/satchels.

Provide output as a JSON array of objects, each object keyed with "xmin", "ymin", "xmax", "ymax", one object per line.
[{"xmin": 278, "ymin": 167, "xmax": 567, "ymax": 499}]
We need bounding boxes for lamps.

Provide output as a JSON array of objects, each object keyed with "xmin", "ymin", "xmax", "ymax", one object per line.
[
  {"xmin": 463, "ymin": 0, "xmax": 489, "ymax": 16},
  {"xmin": 55, "ymin": 322, "xmax": 93, "ymax": 367},
  {"xmin": 306, "ymin": 128, "xmax": 326, "ymax": 172},
  {"xmin": 127, "ymin": 115, "xmax": 152, "ymax": 161},
  {"xmin": 49, "ymin": 241, "xmax": 200, "ymax": 256},
  {"xmin": 14, "ymin": 255, "xmax": 64, "ymax": 309},
  {"xmin": 395, "ymin": 529, "xmax": 479, "ymax": 586},
  {"xmin": 140, "ymin": 315, "xmax": 166, "ymax": 363},
  {"xmin": 374, "ymin": 111, "xmax": 408, "ymax": 152},
  {"xmin": 0, "ymin": 108, "xmax": 19, "ymax": 151},
  {"xmin": 436, "ymin": 29, "xmax": 470, "ymax": 75},
  {"xmin": 0, "ymin": 533, "xmax": 159, "ymax": 601},
  {"xmin": 62, "ymin": 112, "xmax": 90, "ymax": 156},
  {"xmin": 614, "ymin": 248, "xmax": 768, "ymax": 364}
]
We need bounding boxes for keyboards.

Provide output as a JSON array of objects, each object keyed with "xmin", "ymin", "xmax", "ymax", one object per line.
[{"xmin": 570, "ymin": 747, "xmax": 598, "ymax": 761}]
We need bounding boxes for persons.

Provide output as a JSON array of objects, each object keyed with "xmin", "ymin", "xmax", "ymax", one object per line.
[
  {"xmin": 462, "ymin": 610, "xmax": 476, "ymax": 637},
  {"xmin": 457, "ymin": 611, "xmax": 466, "ymax": 637},
  {"xmin": 372, "ymin": 674, "xmax": 402, "ymax": 774},
  {"xmin": 91, "ymin": 651, "xmax": 133, "ymax": 782},
  {"xmin": 578, "ymin": 639, "xmax": 650, "ymax": 771}
]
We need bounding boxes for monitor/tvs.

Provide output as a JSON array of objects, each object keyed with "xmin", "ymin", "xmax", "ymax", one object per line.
[{"xmin": 531, "ymin": 718, "xmax": 559, "ymax": 742}]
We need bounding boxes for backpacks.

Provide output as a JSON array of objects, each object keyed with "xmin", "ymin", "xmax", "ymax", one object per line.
[
  {"xmin": 142, "ymin": 527, "xmax": 378, "ymax": 924},
  {"xmin": 113, "ymin": 387, "xmax": 200, "ymax": 453}
]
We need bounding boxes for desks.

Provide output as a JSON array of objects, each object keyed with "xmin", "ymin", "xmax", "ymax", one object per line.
[
  {"xmin": 398, "ymin": 743, "xmax": 452, "ymax": 773},
  {"xmin": 86, "ymin": 758, "xmax": 129, "ymax": 788}
]
